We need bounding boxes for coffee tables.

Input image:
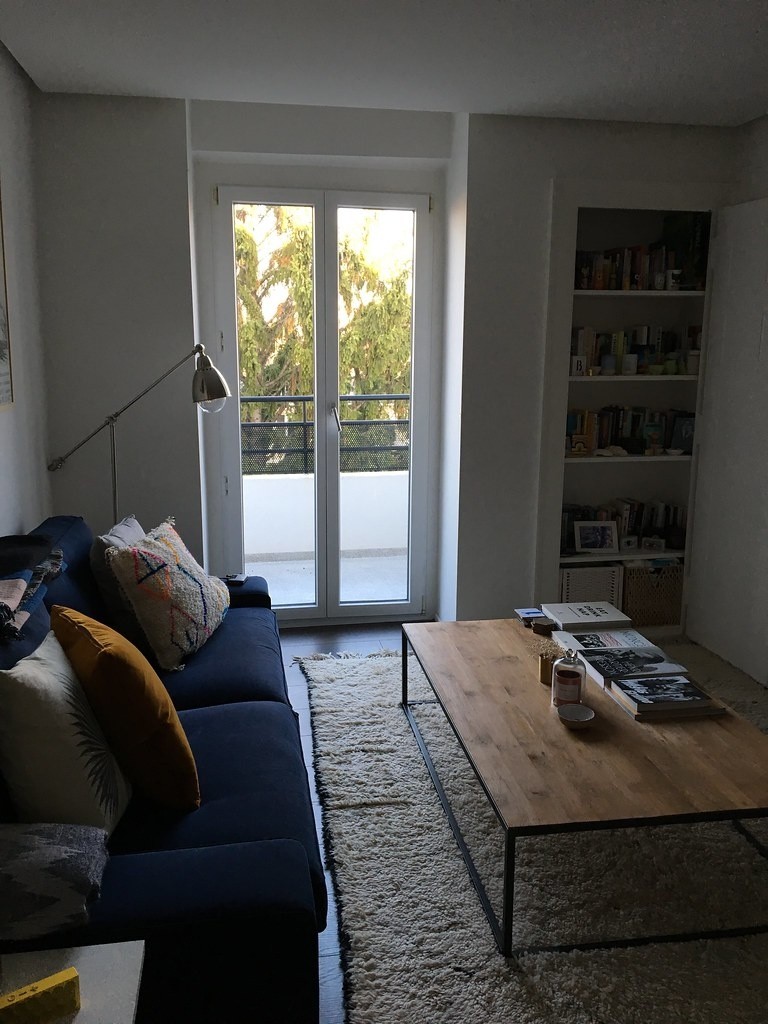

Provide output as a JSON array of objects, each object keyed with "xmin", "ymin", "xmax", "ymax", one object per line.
[{"xmin": 397, "ymin": 620, "xmax": 766, "ymax": 958}]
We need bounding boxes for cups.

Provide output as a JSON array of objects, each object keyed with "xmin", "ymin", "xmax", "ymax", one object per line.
[
  {"xmin": 602, "ymin": 355, "xmax": 615, "ymax": 376},
  {"xmin": 539, "ymin": 653, "xmax": 559, "ymax": 685},
  {"xmin": 667, "ymin": 270, "xmax": 681, "ymax": 290},
  {"xmin": 622, "ymin": 354, "xmax": 637, "ymax": 375}
]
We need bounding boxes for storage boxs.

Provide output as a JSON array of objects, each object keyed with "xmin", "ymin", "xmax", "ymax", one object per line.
[
  {"xmin": 558, "ymin": 559, "xmax": 622, "ymax": 613},
  {"xmin": 623, "ymin": 565, "xmax": 681, "ymax": 627}
]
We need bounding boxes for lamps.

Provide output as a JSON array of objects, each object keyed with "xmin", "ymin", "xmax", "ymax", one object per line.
[{"xmin": 46, "ymin": 343, "xmax": 232, "ymax": 528}]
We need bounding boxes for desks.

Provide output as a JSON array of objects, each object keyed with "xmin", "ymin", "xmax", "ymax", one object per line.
[{"xmin": 0, "ymin": 940, "xmax": 145, "ymax": 1024}]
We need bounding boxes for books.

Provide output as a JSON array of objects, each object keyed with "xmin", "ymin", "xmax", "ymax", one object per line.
[
  {"xmin": 563, "ymin": 241, "xmax": 708, "ymax": 560},
  {"xmin": 541, "ymin": 602, "xmax": 726, "ymax": 722}
]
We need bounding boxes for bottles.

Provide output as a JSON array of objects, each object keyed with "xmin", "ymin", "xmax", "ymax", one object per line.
[{"xmin": 551, "ymin": 648, "xmax": 586, "ymax": 707}]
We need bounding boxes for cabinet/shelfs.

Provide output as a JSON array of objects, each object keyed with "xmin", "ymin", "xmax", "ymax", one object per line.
[{"xmin": 528, "ymin": 175, "xmax": 717, "ymax": 640}]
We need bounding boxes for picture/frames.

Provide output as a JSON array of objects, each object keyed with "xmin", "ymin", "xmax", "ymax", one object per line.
[{"xmin": 573, "ymin": 521, "xmax": 620, "ymax": 555}]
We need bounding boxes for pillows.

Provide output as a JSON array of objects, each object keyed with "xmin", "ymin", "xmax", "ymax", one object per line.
[
  {"xmin": 106, "ymin": 519, "xmax": 231, "ymax": 677},
  {"xmin": 93, "ymin": 513, "xmax": 149, "ymax": 660},
  {"xmin": 0, "ymin": 630, "xmax": 131, "ymax": 847},
  {"xmin": 46, "ymin": 602, "xmax": 203, "ymax": 811}
]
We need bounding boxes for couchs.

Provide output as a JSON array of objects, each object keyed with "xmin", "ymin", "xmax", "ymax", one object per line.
[{"xmin": 0, "ymin": 505, "xmax": 328, "ymax": 1024}]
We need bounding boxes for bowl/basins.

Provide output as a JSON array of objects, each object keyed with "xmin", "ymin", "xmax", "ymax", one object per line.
[
  {"xmin": 665, "ymin": 449, "xmax": 684, "ymax": 455},
  {"xmin": 556, "ymin": 704, "xmax": 595, "ymax": 730}
]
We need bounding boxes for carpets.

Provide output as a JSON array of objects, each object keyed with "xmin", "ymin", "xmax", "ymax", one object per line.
[{"xmin": 298, "ymin": 648, "xmax": 768, "ymax": 1024}]
